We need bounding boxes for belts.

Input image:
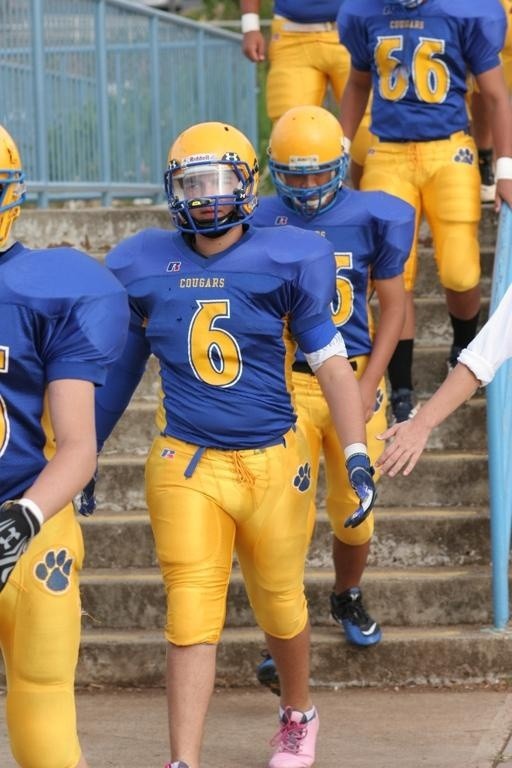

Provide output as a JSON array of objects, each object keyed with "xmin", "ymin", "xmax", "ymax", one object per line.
[
  {"xmin": 379, "ymin": 134, "xmax": 448, "ymax": 143},
  {"xmin": 292, "ymin": 361, "xmax": 358, "ymax": 376},
  {"xmin": 281, "ymin": 13, "xmax": 337, "ymax": 34}
]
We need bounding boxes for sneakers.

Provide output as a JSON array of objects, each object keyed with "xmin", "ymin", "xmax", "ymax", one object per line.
[
  {"xmin": 445, "ymin": 344, "xmax": 487, "ymax": 407},
  {"xmin": 328, "ymin": 584, "xmax": 382, "ymax": 648},
  {"xmin": 255, "ymin": 648, "xmax": 283, "ymax": 698},
  {"xmin": 385, "ymin": 388, "xmax": 418, "ymax": 444},
  {"xmin": 477, "ymin": 155, "xmax": 496, "ymax": 204},
  {"xmin": 267, "ymin": 700, "xmax": 320, "ymax": 768}
]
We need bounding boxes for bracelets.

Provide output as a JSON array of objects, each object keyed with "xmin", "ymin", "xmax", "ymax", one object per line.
[
  {"xmin": 241, "ymin": 10, "xmax": 265, "ymax": 36},
  {"xmin": 494, "ymin": 154, "xmax": 511, "ymax": 181}
]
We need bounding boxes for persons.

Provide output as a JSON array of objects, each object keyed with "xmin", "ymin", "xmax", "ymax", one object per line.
[
  {"xmin": 3, "ymin": 123, "xmax": 130, "ymax": 768},
  {"xmin": 459, "ymin": 0, "xmax": 510, "ymax": 209},
  {"xmin": 329, "ymin": 0, "xmax": 510, "ymax": 420},
  {"xmin": 75, "ymin": 122, "xmax": 375, "ymax": 768},
  {"xmin": 249, "ymin": 103, "xmax": 416, "ymax": 702},
  {"xmin": 239, "ymin": 0, "xmax": 375, "ymax": 195},
  {"xmin": 370, "ymin": 267, "xmax": 512, "ymax": 480}
]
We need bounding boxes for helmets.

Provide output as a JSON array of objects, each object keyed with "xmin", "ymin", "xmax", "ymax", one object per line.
[
  {"xmin": 164, "ymin": 121, "xmax": 258, "ymax": 234},
  {"xmin": 0, "ymin": 125, "xmax": 26, "ymax": 250},
  {"xmin": 266, "ymin": 104, "xmax": 351, "ymax": 212}
]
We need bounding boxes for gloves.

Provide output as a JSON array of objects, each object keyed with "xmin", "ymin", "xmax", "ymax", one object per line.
[
  {"xmin": 0, "ymin": 497, "xmax": 45, "ymax": 594},
  {"xmin": 342, "ymin": 442, "xmax": 378, "ymax": 528},
  {"xmin": 74, "ymin": 467, "xmax": 99, "ymax": 520}
]
